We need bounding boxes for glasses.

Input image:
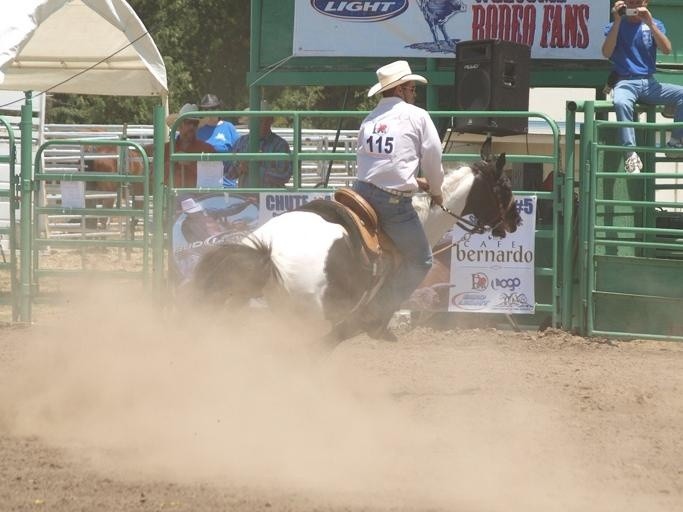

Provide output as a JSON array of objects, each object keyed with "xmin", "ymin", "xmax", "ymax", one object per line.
[{"xmin": 402, "ymin": 85, "xmax": 417, "ymax": 95}]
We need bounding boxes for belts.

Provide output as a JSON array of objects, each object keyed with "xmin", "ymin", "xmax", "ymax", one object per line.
[{"xmin": 374, "ymin": 185, "xmax": 413, "ymax": 198}]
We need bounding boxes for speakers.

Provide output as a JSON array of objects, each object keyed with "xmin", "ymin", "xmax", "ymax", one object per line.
[
  {"xmin": 451, "ymin": 39, "xmax": 531, "ymax": 137},
  {"xmin": 511, "ymin": 163, "xmax": 543, "ymax": 221}
]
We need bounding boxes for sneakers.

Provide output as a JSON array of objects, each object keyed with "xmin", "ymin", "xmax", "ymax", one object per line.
[
  {"xmin": 354, "ymin": 307, "xmax": 398, "ymax": 342},
  {"xmin": 624, "ymin": 153, "xmax": 643, "ymax": 174},
  {"xmin": 664, "ymin": 142, "xmax": 683, "ymax": 159}
]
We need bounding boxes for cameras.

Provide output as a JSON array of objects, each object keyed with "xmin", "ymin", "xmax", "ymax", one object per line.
[{"xmin": 616, "ymin": 3, "xmax": 639, "ymax": 16}]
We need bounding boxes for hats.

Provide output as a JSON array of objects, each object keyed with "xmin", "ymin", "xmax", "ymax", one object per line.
[
  {"xmin": 181, "ymin": 197, "xmax": 202, "ymax": 214},
  {"xmin": 238, "ymin": 99, "xmax": 279, "ymax": 125},
  {"xmin": 166, "ymin": 103, "xmax": 209, "ymax": 132},
  {"xmin": 367, "ymin": 60, "xmax": 429, "ymax": 98},
  {"xmin": 197, "ymin": 94, "xmax": 225, "ymax": 108}
]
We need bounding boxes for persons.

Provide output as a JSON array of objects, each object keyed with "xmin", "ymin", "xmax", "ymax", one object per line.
[
  {"xmin": 176, "ymin": 93, "xmax": 241, "ymax": 188},
  {"xmin": 602, "ymin": 0, "xmax": 683, "ymax": 174},
  {"xmin": 150, "ymin": 102, "xmax": 217, "ymax": 232},
  {"xmin": 351, "ymin": 60, "xmax": 445, "ymax": 342},
  {"xmin": 224, "ymin": 99, "xmax": 292, "ymax": 188}
]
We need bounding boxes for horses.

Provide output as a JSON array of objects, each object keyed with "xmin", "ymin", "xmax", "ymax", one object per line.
[
  {"xmin": 191, "ymin": 136, "xmax": 524, "ymax": 350},
  {"xmin": 79, "ymin": 142, "xmax": 173, "ymax": 260}
]
